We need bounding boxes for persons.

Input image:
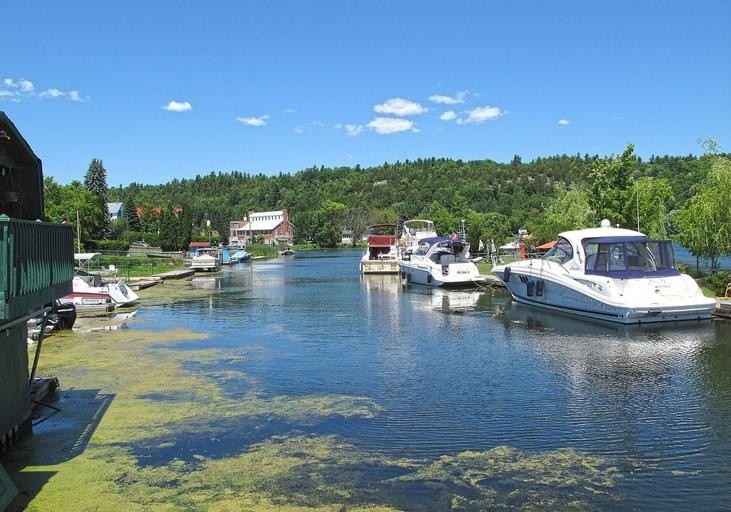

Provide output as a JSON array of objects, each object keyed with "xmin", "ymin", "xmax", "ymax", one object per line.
[{"xmin": 450, "ymin": 231, "xmax": 460, "ymax": 255}]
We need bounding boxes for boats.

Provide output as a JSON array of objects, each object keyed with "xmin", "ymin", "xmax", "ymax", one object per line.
[
  {"xmin": 407, "ymin": 284, "xmax": 485, "ymax": 317},
  {"xmin": 68, "ymin": 310, "xmax": 141, "ymax": 332},
  {"xmin": 56, "ymin": 252, "xmax": 140, "ymax": 309},
  {"xmin": 232, "ymin": 263, "xmax": 250, "ymax": 270},
  {"xmin": 189, "ymin": 246, "xmax": 224, "ymax": 272},
  {"xmin": 192, "ymin": 274, "xmax": 225, "ymax": 295},
  {"xmin": 358, "ymin": 222, "xmax": 402, "ymax": 273},
  {"xmin": 490, "ymin": 218, "xmax": 717, "ymax": 325},
  {"xmin": 490, "ymin": 299, "xmax": 716, "ymax": 395},
  {"xmin": 359, "ymin": 275, "xmax": 402, "ymax": 295},
  {"xmin": 277, "ymin": 248, "xmax": 295, "ymax": 256},
  {"xmin": 396, "ymin": 236, "xmax": 486, "ymax": 289},
  {"xmin": 230, "ymin": 250, "xmax": 252, "ymax": 262},
  {"xmin": 398, "ymin": 220, "xmax": 438, "ymax": 259}
]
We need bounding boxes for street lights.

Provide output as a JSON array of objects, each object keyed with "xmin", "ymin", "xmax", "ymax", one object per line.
[
  {"xmin": 243, "ymin": 213, "xmax": 247, "ymax": 238},
  {"xmin": 206, "ymin": 220, "xmax": 211, "ymax": 245}
]
